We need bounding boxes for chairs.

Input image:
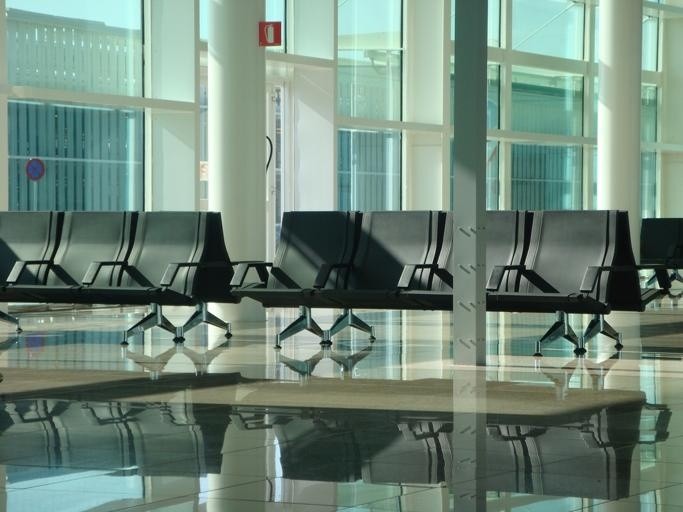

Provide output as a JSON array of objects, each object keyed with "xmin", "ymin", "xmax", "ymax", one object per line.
[
  {"xmin": 0, "ymin": 396, "xmax": 674, "ymax": 500},
  {"xmin": 0, "ymin": 209, "xmax": 264, "ymax": 348},
  {"xmin": 229, "ymin": 211, "xmax": 683, "ymax": 360}
]
